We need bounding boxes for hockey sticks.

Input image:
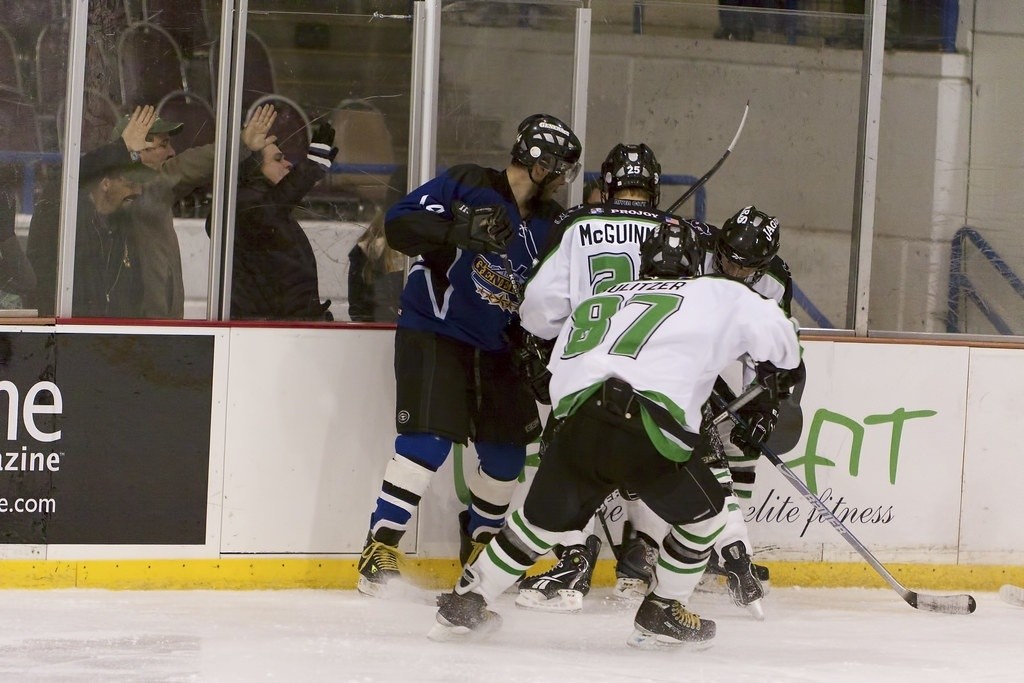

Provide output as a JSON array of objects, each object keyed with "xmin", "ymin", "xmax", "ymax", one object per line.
[
  {"xmin": 499, "ymin": 253, "xmax": 622, "ymax": 561},
  {"xmin": 710, "ymin": 386, "xmax": 976, "ymax": 615},
  {"xmin": 666, "ymin": 96, "xmax": 751, "ymax": 215},
  {"xmin": 700, "ymin": 381, "xmax": 769, "ymax": 436}
]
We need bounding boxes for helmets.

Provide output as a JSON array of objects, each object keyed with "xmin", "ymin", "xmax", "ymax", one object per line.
[
  {"xmin": 639, "ymin": 222, "xmax": 702, "ymax": 280},
  {"xmin": 510, "ymin": 113, "xmax": 582, "ymax": 167},
  {"xmin": 715, "ymin": 206, "xmax": 780, "ymax": 283},
  {"xmin": 601, "ymin": 142, "xmax": 663, "ymax": 210}
]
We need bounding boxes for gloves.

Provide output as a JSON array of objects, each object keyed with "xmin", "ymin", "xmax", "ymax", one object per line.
[
  {"xmin": 307, "ymin": 122, "xmax": 339, "ymax": 181},
  {"xmin": 447, "ymin": 206, "xmax": 515, "ymax": 256},
  {"xmin": 730, "ymin": 361, "xmax": 805, "ymax": 459}
]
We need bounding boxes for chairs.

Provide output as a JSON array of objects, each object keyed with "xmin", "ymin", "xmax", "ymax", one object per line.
[{"xmin": 0, "ymin": 0, "xmax": 396, "ymax": 215}]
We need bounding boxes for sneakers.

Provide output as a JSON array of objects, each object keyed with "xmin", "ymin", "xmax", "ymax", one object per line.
[
  {"xmin": 627, "ymin": 569, "xmax": 716, "ymax": 651},
  {"xmin": 458, "ymin": 511, "xmax": 527, "ymax": 596},
  {"xmin": 693, "ymin": 546, "xmax": 770, "ymax": 594},
  {"xmin": 427, "ymin": 585, "xmax": 503, "ymax": 643},
  {"xmin": 721, "ymin": 541, "xmax": 765, "ymax": 620},
  {"xmin": 513, "ymin": 534, "xmax": 602, "ymax": 614},
  {"xmin": 615, "ymin": 521, "xmax": 660, "ymax": 604},
  {"xmin": 358, "ymin": 512, "xmax": 404, "ymax": 598}
]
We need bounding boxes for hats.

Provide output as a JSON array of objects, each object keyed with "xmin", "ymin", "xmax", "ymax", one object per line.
[
  {"xmin": 109, "ymin": 111, "xmax": 184, "ymax": 140},
  {"xmin": 78, "ymin": 137, "xmax": 141, "ymax": 183}
]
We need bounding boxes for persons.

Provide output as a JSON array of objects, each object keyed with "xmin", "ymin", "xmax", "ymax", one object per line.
[
  {"xmin": 0, "ymin": 102, "xmax": 340, "ymax": 321},
  {"xmin": 348, "ymin": 113, "xmax": 807, "ymax": 652}
]
